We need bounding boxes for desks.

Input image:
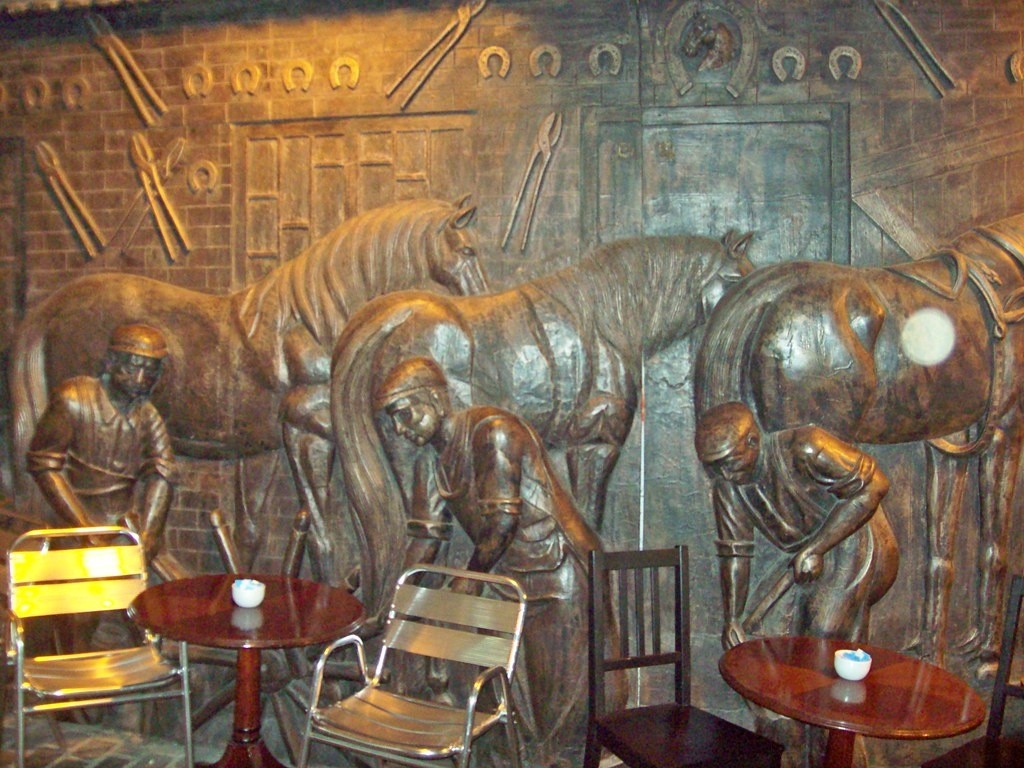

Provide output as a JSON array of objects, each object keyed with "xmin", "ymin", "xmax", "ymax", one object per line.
[
  {"xmin": 718, "ymin": 636, "xmax": 986, "ymax": 768},
  {"xmin": 126, "ymin": 575, "xmax": 368, "ymax": 768}
]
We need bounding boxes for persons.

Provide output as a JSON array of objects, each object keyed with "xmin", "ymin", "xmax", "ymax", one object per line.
[
  {"xmin": 693, "ymin": 401, "xmax": 899, "ymax": 768},
  {"xmin": 375, "ymin": 357, "xmax": 611, "ymax": 768},
  {"xmin": 25, "ymin": 324, "xmax": 180, "ymax": 723}
]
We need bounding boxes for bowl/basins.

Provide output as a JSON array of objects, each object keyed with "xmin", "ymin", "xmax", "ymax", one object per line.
[
  {"xmin": 231, "ymin": 582, "xmax": 266, "ymax": 608},
  {"xmin": 834, "ymin": 649, "xmax": 872, "ymax": 681}
]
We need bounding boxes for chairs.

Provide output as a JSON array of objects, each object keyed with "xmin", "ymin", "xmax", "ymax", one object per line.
[
  {"xmin": 918, "ymin": 574, "xmax": 1024, "ymax": 768},
  {"xmin": 2, "ymin": 525, "xmax": 193, "ymax": 768},
  {"xmin": 583, "ymin": 545, "xmax": 785, "ymax": 768},
  {"xmin": 298, "ymin": 564, "xmax": 528, "ymax": 768}
]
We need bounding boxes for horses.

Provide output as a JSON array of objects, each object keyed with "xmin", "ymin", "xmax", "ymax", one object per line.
[
  {"xmin": 3, "ymin": 194, "xmax": 492, "ymax": 697},
  {"xmin": 328, "ymin": 210, "xmax": 1023, "ymax": 685}
]
